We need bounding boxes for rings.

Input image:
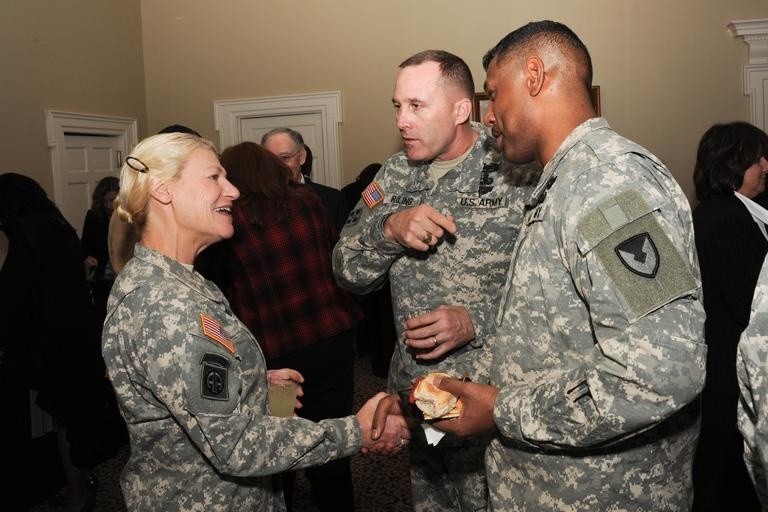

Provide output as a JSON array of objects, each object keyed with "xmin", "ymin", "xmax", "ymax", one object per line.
[{"xmin": 433, "ymin": 336, "xmax": 439, "ymax": 346}]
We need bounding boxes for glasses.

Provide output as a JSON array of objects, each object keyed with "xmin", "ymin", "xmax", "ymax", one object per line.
[{"xmin": 279, "ymin": 149, "xmax": 301, "ymax": 161}]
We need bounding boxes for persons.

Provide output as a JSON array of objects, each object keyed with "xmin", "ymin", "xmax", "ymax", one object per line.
[
  {"xmin": 361, "ymin": 20, "xmax": 708, "ymax": 512},
  {"xmin": 688, "ymin": 121, "xmax": 768, "ymax": 512},
  {"xmin": 423, "ymin": 231, "xmax": 432, "ymax": 244},
  {"xmin": 332, "ymin": 49, "xmax": 543, "ymax": 512}
]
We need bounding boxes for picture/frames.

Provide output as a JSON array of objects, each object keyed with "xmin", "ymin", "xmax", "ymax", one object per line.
[{"xmin": 470, "ymin": 83, "xmax": 604, "ymax": 126}]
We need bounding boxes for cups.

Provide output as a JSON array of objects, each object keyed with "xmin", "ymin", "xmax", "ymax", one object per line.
[{"xmin": 268, "ymin": 370, "xmax": 300, "ymax": 418}]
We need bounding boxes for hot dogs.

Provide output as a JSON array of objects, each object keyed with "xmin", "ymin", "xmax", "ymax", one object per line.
[{"xmin": 408, "ymin": 372, "xmax": 460, "ymax": 419}]
